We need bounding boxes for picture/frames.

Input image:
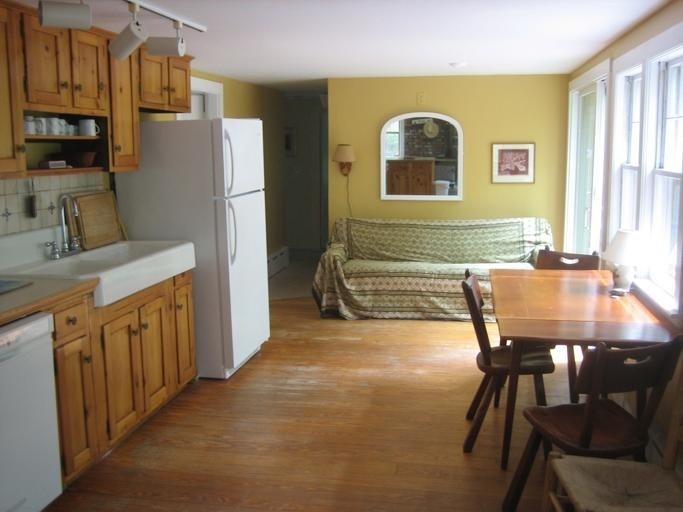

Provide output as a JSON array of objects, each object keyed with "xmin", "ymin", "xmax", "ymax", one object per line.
[{"xmin": 491, "ymin": 143, "xmax": 535, "ymax": 184}]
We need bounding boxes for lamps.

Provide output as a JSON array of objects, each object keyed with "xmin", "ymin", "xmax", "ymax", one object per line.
[
  {"xmin": 107, "ymin": 20, "xmax": 149, "ymax": 62},
  {"xmin": 37, "ymin": 1, "xmax": 92, "ymax": 30},
  {"xmin": 333, "ymin": 143, "xmax": 356, "ymax": 177},
  {"xmin": 145, "ymin": 36, "xmax": 186, "ymax": 57}
]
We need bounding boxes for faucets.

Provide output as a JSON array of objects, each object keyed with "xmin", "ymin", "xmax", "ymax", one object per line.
[{"xmin": 44, "ymin": 193, "xmax": 82, "ymax": 260}]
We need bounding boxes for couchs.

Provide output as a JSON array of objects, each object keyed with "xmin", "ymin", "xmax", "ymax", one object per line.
[{"xmin": 311, "ymin": 216, "xmax": 555, "ymax": 324}]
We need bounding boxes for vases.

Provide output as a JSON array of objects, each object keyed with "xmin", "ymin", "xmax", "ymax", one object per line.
[{"xmin": 612, "ymin": 229, "xmax": 637, "ymax": 293}]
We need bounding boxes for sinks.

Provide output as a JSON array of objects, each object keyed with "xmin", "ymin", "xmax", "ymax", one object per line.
[{"xmin": 3, "ymin": 240, "xmax": 196, "ymax": 307}]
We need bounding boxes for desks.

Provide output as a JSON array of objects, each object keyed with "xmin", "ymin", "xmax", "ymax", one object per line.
[{"xmin": 489, "ymin": 265, "xmax": 682, "ymax": 470}]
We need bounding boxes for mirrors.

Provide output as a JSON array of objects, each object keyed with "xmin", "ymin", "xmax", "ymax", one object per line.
[{"xmin": 379, "ymin": 111, "xmax": 463, "ymax": 202}]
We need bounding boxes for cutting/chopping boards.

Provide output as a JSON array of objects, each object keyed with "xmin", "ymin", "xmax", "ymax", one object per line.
[{"xmin": 71, "ymin": 189, "xmax": 124, "ymax": 250}]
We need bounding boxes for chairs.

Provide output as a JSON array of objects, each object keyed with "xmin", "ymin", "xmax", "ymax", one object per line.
[
  {"xmin": 536, "ymin": 250, "xmax": 601, "ymax": 272},
  {"xmin": 501, "ymin": 334, "xmax": 683, "ymax": 512},
  {"xmin": 541, "ymin": 367, "xmax": 683, "ymax": 512},
  {"xmin": 462, "ymin": 268, "xmax": 555, "ymax": 462}
]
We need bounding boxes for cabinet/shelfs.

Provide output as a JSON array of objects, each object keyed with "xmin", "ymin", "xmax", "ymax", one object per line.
[
  {"xmin": 20, "ymin": 13, "xmax": 113, "ymax": 177},
  {"xmin": 53, "ymin": 298, "xmax": 101, "ymax": 478},
  {"xmin": 107, "ymin": 38, "xmax": 140, "ymax": 173},
  {"xmin": 0, "ymin": 8, "xmax": 22, "ymax": 180},
  {"xmin": 139, "ymin": 49, "xmax": 193, "ymax": 114},
  {"xmin": 102, "ymin": 296, "xmax": 175, "ymax": 440},
  {"xmin": 174, "ymin": 283, "xmax": 197, "ymax": 384}
]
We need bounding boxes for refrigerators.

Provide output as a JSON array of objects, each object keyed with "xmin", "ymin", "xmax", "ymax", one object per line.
[{"xmin": 115, "ymin": 117, "xmax": 271, "ymax": 379}]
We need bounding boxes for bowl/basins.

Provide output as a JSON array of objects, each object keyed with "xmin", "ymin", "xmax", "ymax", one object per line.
[{"xmin": 73, "ymin": 151, "xmax": 96, "ymax": 167}]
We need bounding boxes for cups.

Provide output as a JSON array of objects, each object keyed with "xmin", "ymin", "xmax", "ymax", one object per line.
[
  {"xmin": 59, "ymin": 119, "xmax": 65, "ymax": 136},
  {"xmin": 35, "ymin": 117, "xmax": 47, "ymax": 135},
  {"xmin": 79, "ymin": 119, "xmax": 101, "ymax": 137},
  {"xmin": 47, "ymin": 117, "xmax": 62, "ymax": 136}
]
